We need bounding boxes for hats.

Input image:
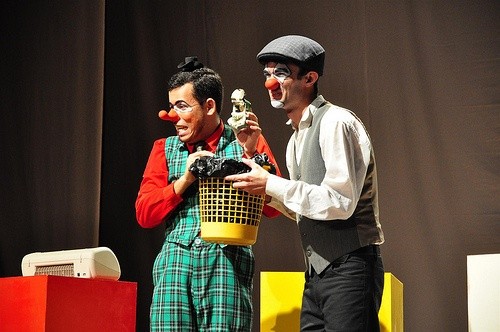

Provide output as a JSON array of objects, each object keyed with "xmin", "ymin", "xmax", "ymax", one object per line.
[{"xmin": 256, "ymin": 35, "xmax": 326, "ymax": 76}]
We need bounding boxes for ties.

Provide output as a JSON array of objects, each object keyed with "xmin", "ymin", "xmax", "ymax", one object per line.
[{"xmin": 192, "ymin": 140, "xmax": 208, "ymax": 153}]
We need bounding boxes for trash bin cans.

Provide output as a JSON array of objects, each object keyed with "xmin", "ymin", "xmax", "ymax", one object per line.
[{"xmin": 193, "ymin": 156, "xmax": 272, "ymax": 246}]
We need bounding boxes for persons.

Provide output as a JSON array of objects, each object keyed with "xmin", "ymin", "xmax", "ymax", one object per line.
[
  {"xmin": 225, "ymin": 34, "xmax": 385, "ymax": 332},
  {"xmin": 135, "ymin": 56, "xmax": 283, "ymax": 332}
]
